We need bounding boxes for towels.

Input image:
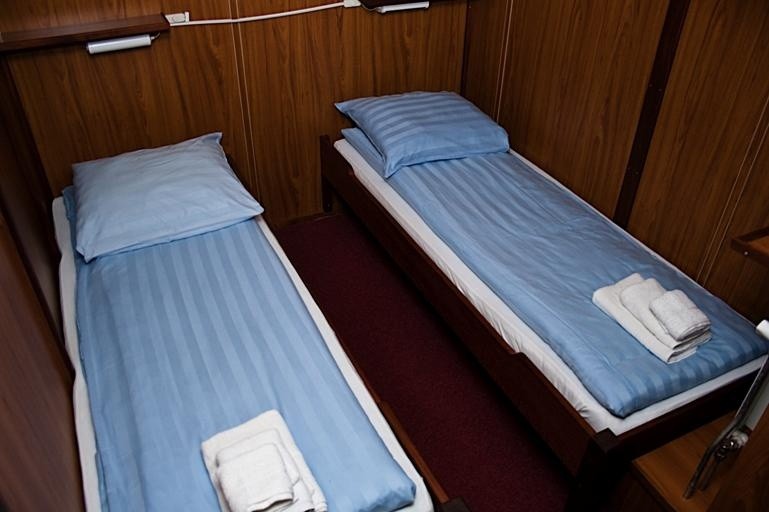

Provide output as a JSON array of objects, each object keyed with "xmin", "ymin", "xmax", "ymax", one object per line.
[
  {"xmin": 201, "ymin": 409, "xmax": 328, "ymax": 512},
  {"xmin": 592, "ymin": 272, "xmax": 712, "ymax": 365}
]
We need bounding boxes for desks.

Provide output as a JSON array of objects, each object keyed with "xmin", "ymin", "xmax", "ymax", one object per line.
[{"xmin": 613, "ymin": 401, "xmax": 769, "ymax": 512}]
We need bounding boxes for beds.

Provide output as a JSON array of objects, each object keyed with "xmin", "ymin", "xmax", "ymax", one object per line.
[
  {"xmin": 53, "ymin": 133, "xmax": 470, "ymax": 512},
  {"xmin": 320, "ymin": 92, "xmax": 769, "ymax": 512}
]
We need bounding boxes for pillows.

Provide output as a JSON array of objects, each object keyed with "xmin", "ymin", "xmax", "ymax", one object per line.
[
  {"xmin": 335, "ymin": 92, "xmax": 512, "ymax": 178},
  {"xmin": 70, "ymin": 132, "xmax": 265, "ymax": 264}
]
